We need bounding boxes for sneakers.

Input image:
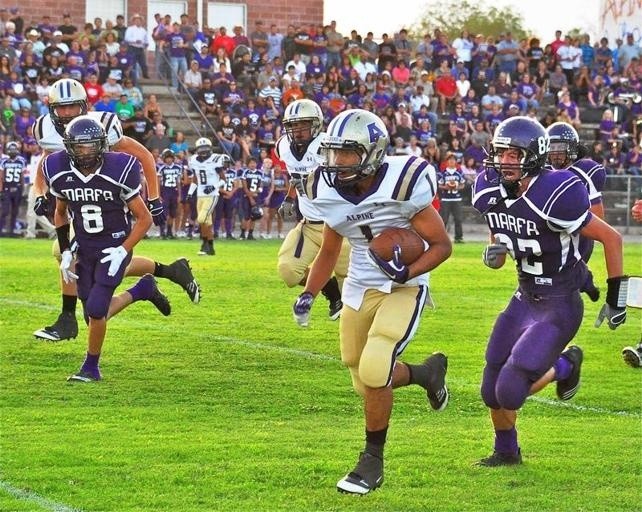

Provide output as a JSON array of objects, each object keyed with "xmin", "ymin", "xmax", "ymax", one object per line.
[
  {"xmin": 337, "ymin": 452, "xmax": 384, "ymax": 494},
  {"xmin": 477, "ymin": 447, "xmax": 524, "ymax": 467},
  {"xmin": 424, "ymin": 352, "xmax": 448, "ymax": 412},
  {"xmin": 171, "ymin": 258, "xmax": 202, "ymax": 304},
  {"xmin": 623, "ymin": 343, "xmax": 642, "ymax": 367},
  {"xmin": 329, "ymin": 300, "xmax": 344, "ymax": 321},
  {"xmin": 198, "ymin": 236, "xmax": 216, "ymax": 256},
  {"xmin": 67, "ymin": 365, "xmax": 101, "ymax": 382},
  {"xmin": 556, "ymin": 344, "xmax": 582, "ymax": 400},
  {"xmin": 34, "ymin": 313, "xmax": 78, "ymax": 342},
  {"xmin": 141, "ymin": 273, "xmax": 171, "ymax": 314},
  {"xmin": 213, "ymin": 230, "xmax": 285, "ymax": 239},
  {"xmin": 161, "ymin": 230, "xmax": 200, "ymax": 240}
]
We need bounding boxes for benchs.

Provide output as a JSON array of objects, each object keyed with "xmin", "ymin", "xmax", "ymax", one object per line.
[{"xmin": 138, "ymin": 78, "xmax": 642, "ymax": 220}]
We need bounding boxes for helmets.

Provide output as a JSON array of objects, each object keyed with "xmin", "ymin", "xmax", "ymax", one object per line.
[
  {"xmin": 282, "ymin": 98, "xmax": 323, "ymax": 160},
  {"xmin": 196, "ymin": 137, "xmax": 213, "ymax": 157},
  {"xmin": 250, "ymin": 204, "xmax": 263, "ymax": 221},
  {"xmin": 161, "ymin": 148, "xmax": 176, "ymax": 163},
  {"xmin": 5, "ymin": 141, "xmax": 20, "ymax": 159},
  {"xmin": 47, "ymin": 77, "xmax": 88, "ymax": 138},
  {"xmin": 484, "ymin": 113, "xmax": 550, "ymax": 200},
  {"xmin": 63, "ymin": 116, "xmax": 108, "ymax": 170},
  {"xmin": 547, "ymin": 122, "xmax": 580, "ymax": 171},
  {"xmin": 320, "ymin": 109, "xmax": 392, "ymax": 187}
]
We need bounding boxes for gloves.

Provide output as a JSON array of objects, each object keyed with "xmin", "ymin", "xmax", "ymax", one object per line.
[
  {"xmin": 482, "ymin": 244, "xmax": 507, "ymax": 268},
  {"xmin": 595, "ymin": 302, "xmax": 627, "ymax": 329},
  {"xmin": 60, "ymin": 250, "xmax": 80, "ymax": 284},
  {"xmin": 34, "ymin": 196, "xmax": 50, "ymax": 216},
  {"xmin": 367, "ymin": 244, "xmax": 409, "ymax": 285},
  {"xmin": 99, "ymin": 246, "xmax": 128, "ymax": 277},
  {"xmin": 293, "ymin": 292, "xmax": 313, "ymax": 326},
  {"xmin": 147, "ymin": 196, "xmax": 167, "ymax": 227}
]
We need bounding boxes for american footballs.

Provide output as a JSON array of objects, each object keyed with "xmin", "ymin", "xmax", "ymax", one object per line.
[{"xmin": 368, "ymin": 228, "xmax": 425, "ymax": 266}]
